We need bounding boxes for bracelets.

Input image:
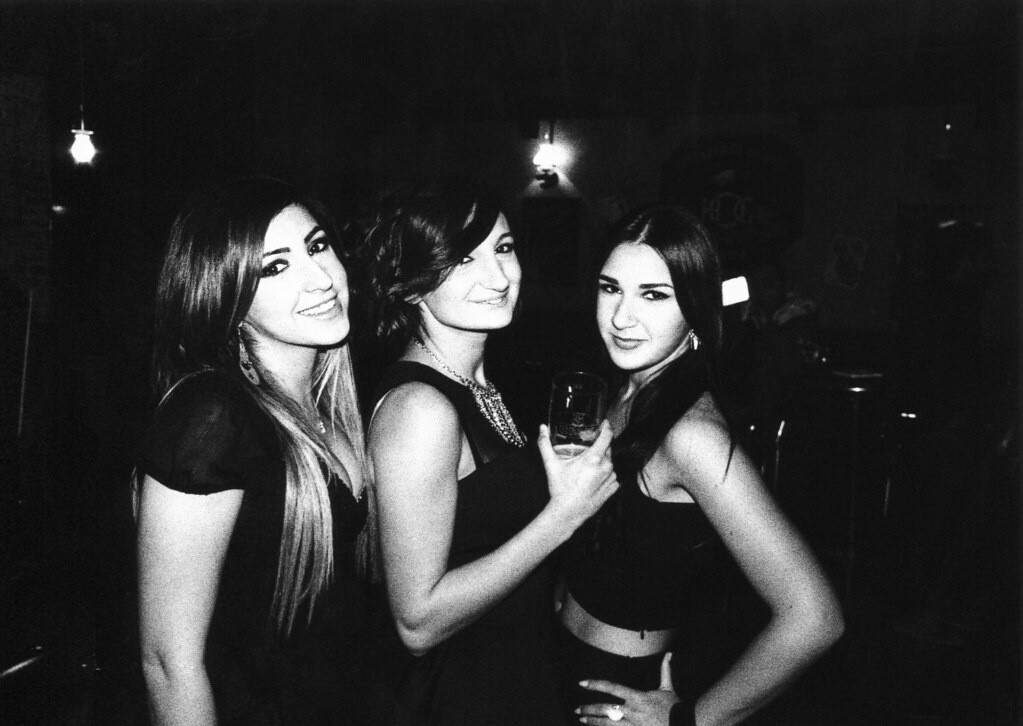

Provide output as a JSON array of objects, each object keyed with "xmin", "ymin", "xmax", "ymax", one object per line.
[{"xmin": 668, "ymin": 700, "xmax": 697, "ymax": 726}]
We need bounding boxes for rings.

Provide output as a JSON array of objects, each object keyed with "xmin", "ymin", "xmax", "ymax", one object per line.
[{"xmin": 609, "ymin": 706, "xmax": 623, "ymax": 722}]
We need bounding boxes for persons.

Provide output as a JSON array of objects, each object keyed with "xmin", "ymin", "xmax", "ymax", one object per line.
[{"xmin": 131, "ymin": 182, "xmax": 846, "ymax": 726}]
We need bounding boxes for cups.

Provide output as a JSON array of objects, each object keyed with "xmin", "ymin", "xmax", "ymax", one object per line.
[{"xmin": 546, "ymin": 371, "xmax": 604, "ymax": 460}]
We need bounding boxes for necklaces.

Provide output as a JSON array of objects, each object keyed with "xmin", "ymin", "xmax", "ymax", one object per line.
[{"xmin": 413, "ymin": 337, "xmax": 526, "ymax": 448}]
